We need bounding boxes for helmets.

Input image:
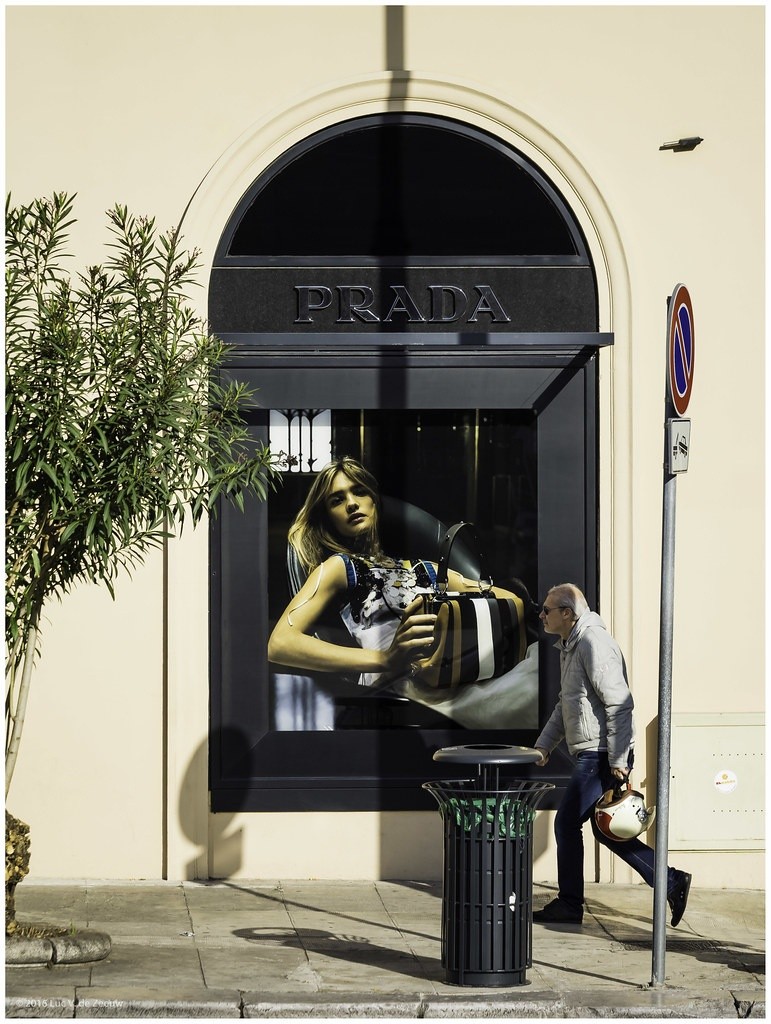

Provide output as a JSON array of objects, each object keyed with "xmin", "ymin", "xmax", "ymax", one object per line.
[{"xmin": 595, "ymin": 789, "xmax": 648, "ymax": 841}]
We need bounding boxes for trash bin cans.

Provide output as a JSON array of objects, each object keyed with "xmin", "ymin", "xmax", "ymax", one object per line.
[{"xmin": 420, "ymin": 743, "xmax": 560, "ymax": 988}]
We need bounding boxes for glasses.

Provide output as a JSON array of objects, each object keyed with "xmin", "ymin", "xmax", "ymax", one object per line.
[{"xmin": 542, "ymin": 604, "xmax": 572, "ymax": 616}]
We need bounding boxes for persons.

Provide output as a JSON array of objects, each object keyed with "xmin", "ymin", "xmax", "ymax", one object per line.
[
  {"xmin": 534, "ymin": 582, "xmax": 693, "ymax": 928},
  {"xmin": 267, "ymin": 458, "xmax": 539, "ymax": 730}
]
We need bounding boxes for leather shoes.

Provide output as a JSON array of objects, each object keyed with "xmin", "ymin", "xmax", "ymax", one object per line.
[
  {"xmin": 532, "ymin": 896, "xmax": 583, "ymax": 924},
  {"xmin": 667, "ymin": 869, "xmax": 692, "ymax": 927}
]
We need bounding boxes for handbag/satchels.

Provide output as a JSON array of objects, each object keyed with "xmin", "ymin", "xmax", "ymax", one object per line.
[{"xmin": 407, "ymin": 587, "xmax": 521, "ymax": 690}]
